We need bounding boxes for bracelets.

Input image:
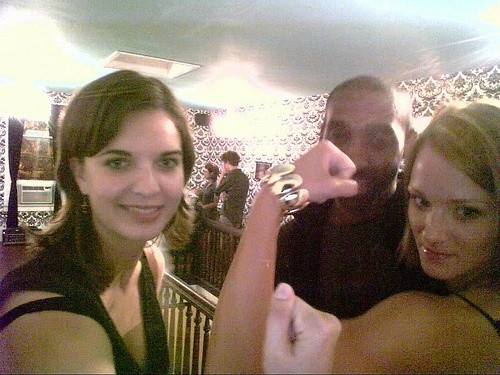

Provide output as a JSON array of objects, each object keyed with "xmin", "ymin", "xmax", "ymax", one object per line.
[{"xmin": 257, "ymin": 161, "xmax": 309, "ymax": 220}]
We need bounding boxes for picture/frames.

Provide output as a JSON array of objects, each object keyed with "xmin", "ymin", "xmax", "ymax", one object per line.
[{"xmin": 254, "ymin": 161, "xmax": 272, "ymax": 181}]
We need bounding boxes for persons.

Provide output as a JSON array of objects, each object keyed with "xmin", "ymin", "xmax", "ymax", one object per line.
[
  {"xmin": 216, "ymin": 150, "xmax": 250, "ymax": 255},
  {"xmin": 273, "ymin": 75, "xmax": 452, "ymax": 324},
  {"xmin": 191, "ymin": 162, "xmax": 220, "ymax": 252},
  {"xmin": 0, "ymin": 68, "xmax": 198, "ymax": 375},
  {"xmin": 203, "ymin": 101, "xmax": 500, "ymax": 375}
]
